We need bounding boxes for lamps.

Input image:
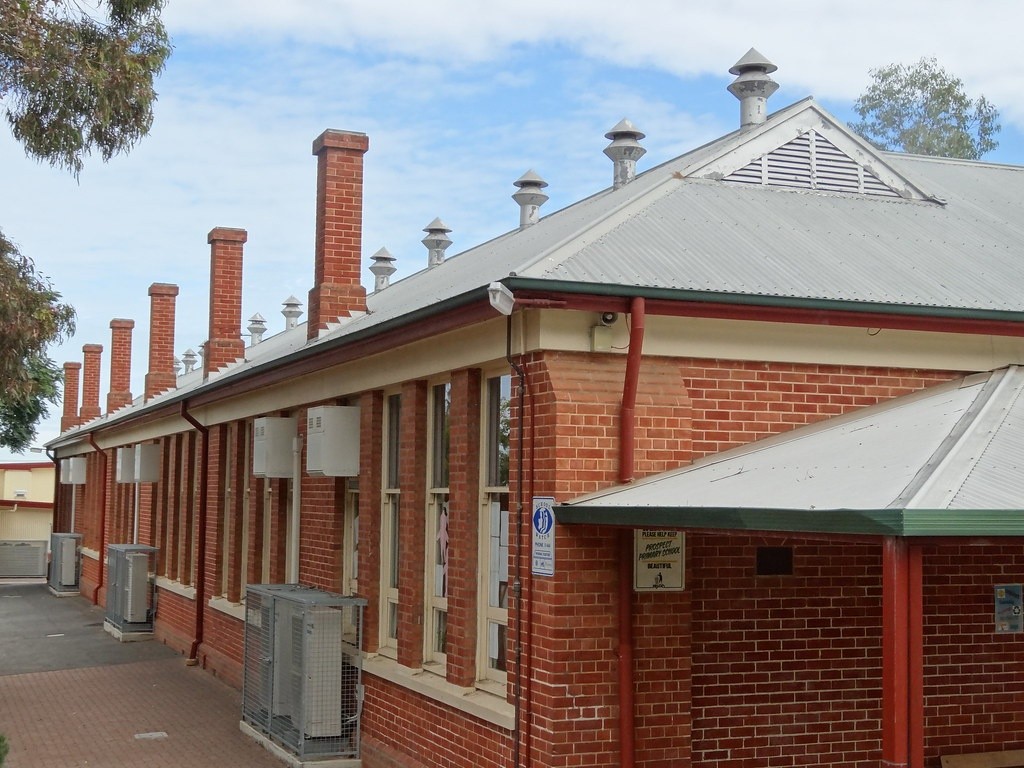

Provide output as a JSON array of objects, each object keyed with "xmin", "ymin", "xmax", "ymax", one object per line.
[
  {"xmin": 29, "ymin": 447, "xmax": 45, "ymax": 453},
  {"xmin": 487, "ymin": 280, "xmax": 516, "ymax": 315}
]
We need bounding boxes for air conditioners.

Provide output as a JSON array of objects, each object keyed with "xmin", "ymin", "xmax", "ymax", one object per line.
[
  {"xmin": 255, "ymin": 591, "xmax": 345, "ymax": 739},
  {"xmin": 108, "ymin": 550, "xmax": 147, "ymax": 623},
  {"xmin": 52, "ymin": 538, "xmax": 75, "ymax": 586}
]
